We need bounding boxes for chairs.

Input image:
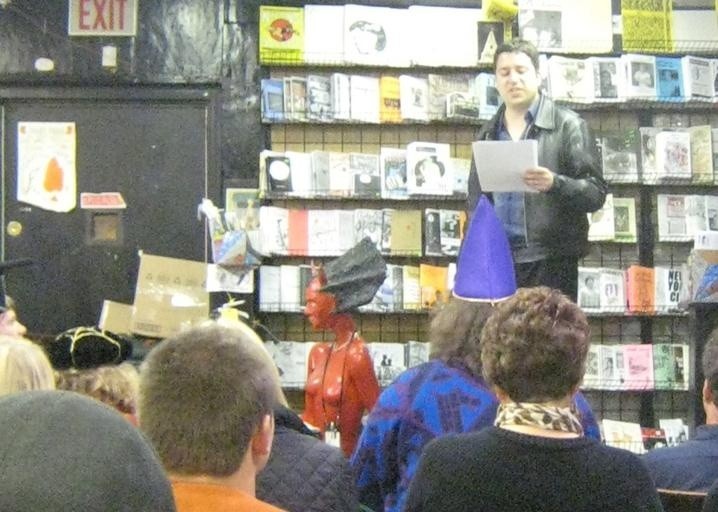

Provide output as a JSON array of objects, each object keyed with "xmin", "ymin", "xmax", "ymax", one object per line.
[{"xmin": 655, "ymin": 487, "xmax": 708, "ymax": 511}]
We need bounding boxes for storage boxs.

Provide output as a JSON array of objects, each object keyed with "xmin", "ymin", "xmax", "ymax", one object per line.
[{"xmin": 132, "ymin": 252, "xmax": 215, "ymax": 348}]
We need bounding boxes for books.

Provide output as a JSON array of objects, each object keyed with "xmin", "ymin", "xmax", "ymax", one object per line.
[
  {"xmin": 408, "ymin": 3, "xmax": 477, "ymax": 66},
  {"xmin": 471, "ymin": 139, "xmax": 541, "ymax": 194},
  {"xmin": 345, "ymin": 5, "xmax": 412, "ymax": 67},
  {"xmin": 131, "ymin": 251, "xmax": 211, "ymax": 338},
  {"xmin": 302, "ymin": 3, "xmax": 347, "ymax": 63},
  {"xmin": 258, "ymin": 5, "xmax": 304, "ymax": 63}
]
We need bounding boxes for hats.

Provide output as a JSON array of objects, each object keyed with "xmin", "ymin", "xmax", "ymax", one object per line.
[
  {"xmin": 450, "ymin": 193, "xmax": 518, "ymax": 303},
  {"xmin": 48, "ymin": 325, "xmax": 135, "ymax": 371}
]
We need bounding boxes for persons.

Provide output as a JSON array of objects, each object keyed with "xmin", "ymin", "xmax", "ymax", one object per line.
[
  {"xmin": 53, "ymin": 361, "xmax": 142, "ymax": 426},
  {"xmin": 640, "ymin": 329, "xmax": 717, "ymax": 494},
  {"xmin": 136, "ymin": 323, "xmax": 289, "ymax": 512},
  {"xmin": 203, "ymin": 318, "xmax": 352, "ymax": 512},
  {"xmin": 462, "ymin": 36, "xmax": 607, "ymax": 303},
  {"xmin": 0, "ymin": 388, "xmax": 175, "ymax": 512},
  {"xmin": 0, "ymin": 296, "xmax": 53, "ymax": 390},
  {"xmin": 351, "ymin": 287, "xmax": 602, "ymax": 512},
  {"xmin": 698, "ymin": 481, "xmax": 717, "ymax": 512},
  {"xmin": 402, "ymin": 285, "xmax": 664, "ymax": 512}
]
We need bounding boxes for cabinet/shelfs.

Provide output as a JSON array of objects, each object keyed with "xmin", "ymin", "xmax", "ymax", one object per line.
[{"xmin": 220, "ymin": 0, "xmax": 717, "ymax": 452}]
